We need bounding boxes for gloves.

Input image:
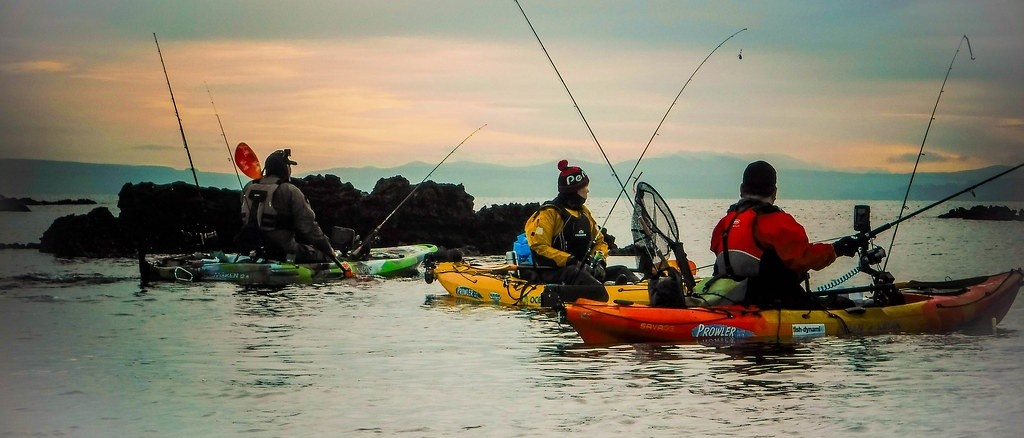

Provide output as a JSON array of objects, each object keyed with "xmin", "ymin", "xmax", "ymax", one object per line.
[
  {"xmin": 593, "ymin": 263, "xmax": 607, "ymax": 284},
  {"xmin": 573, "ymin": 260, "xmax": 594, "ymax": 276},
  {"xmin": 832, "ymin": 232, "xmax": 869, "ymax": 258}
]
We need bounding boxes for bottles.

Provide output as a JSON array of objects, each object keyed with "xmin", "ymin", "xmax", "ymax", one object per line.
[{"xmin": 285, "ymin": 250, "xmax": 296, "ymax": 263}]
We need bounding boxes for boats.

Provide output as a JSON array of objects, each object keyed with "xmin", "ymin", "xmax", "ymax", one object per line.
[
  {"xmin": 138, "ymin": 226, "xmax": 440, "ymax": 287},
  {"xmin": 422, "ymin": 245, "xmax": 698, "ymax": 309},
  {"xmin": 555, "ymin": 264, "xmax": 1024, "ymax": 347}
]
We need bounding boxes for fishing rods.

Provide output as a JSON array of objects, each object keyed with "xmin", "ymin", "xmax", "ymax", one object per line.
[
  {"xmin": 203, "ymin": 80, "xmax": 251, "ymax": 211},
  {"xmin": 352, "ymin": 124, "xmax": 488, "ymax": 258},
  {"xmin": 516, "ymin": 0, "xmax": 749, "ymax": 289},
  {"xmin": 153, "ymin": 32, "xmax": 224, "ymax": 255},
  {"xmin": 835, "ymin": 32, "xmax": 1024, "ymax": 289}
]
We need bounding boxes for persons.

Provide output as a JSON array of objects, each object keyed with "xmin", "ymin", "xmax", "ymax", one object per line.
[
  {"xmin": 240, "ymin": 150, "xmax": 336, "ymax": 263},
  {"xmin": 710, "ymin": 161, "xmax": 858, "ymax": 308},
  {"xmin": 527, "ymin": 160, "xmax": 640, "ymax": 285}
]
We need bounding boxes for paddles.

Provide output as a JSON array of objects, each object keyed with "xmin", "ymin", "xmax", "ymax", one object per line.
[{"xmin": 234, "ymin": 141, "xmax": 357, "ymax": 279}]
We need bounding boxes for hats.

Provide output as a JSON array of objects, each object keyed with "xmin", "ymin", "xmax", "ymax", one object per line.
[
  {"xmin": 265, "ymin": 149, "xmax": 298, "ymax": 184},
  {"xmin": 742, "ymin": 158, "xmax": 776, "ymax": 197},
  {"xmin": 557, "ymin": 160, "xmax": 589, "ymax": 194}
]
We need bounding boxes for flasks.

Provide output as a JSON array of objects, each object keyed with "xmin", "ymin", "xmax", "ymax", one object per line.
[{"xmin": 505, "ymin": 251, "xmax": 520, "ymax": 279}]
